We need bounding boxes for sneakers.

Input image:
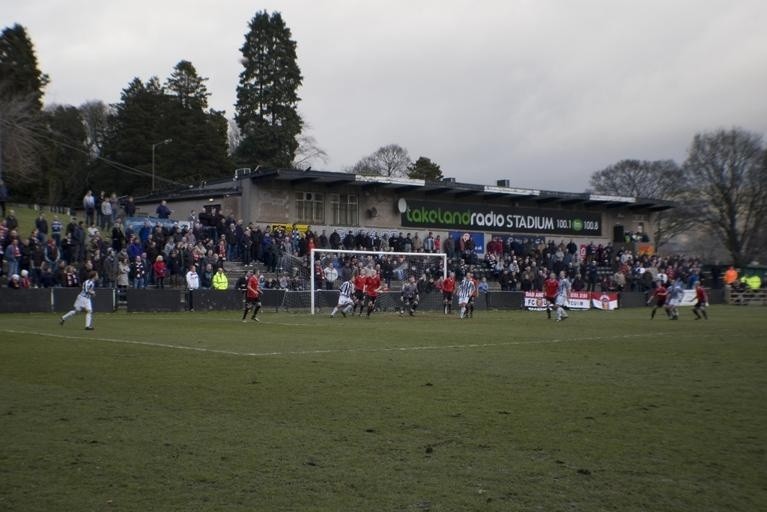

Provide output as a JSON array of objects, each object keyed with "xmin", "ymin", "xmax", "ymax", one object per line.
[
  {"xmin": 11, "ymin": 269, "xmax": 29, "ymax": 280},
  {"xmin": 544, "ymin": 314, "xmax": 568, "ymax": 323},
  {"xmin": 240, "ymin": 317, "xmax": 261, "ymax": 323},
  {"xmin": 329, "ymin": 310, "xmax": 474, "ymax": 320},
  {"xmin": 650, "ymin": 315, "xmax": 708, "ymax": 321},
  {"xmin": 85, "ymin": 326, "xmax": 94, "ymax": 330}
]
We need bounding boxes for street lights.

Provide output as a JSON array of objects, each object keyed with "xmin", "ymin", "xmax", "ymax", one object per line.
[{"xmin": 151, "ymin": 138, "xmax": 173, "ymax": 193}]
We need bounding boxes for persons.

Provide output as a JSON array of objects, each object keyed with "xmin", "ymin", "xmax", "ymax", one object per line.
[{"xmin": 1, "ymin": 188, "xmax": 765, "ymax": 330}]
